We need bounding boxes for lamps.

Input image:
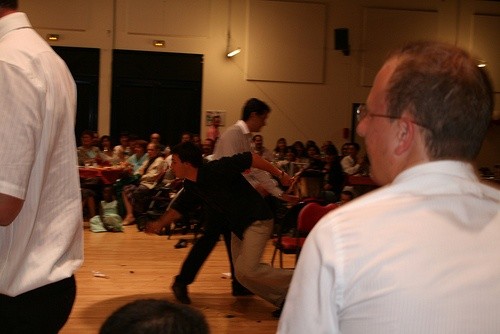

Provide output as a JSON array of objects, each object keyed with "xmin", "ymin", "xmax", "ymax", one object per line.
[{"xmin": 226, "ymin": 47, "xmax": 241, "ymax": 58}]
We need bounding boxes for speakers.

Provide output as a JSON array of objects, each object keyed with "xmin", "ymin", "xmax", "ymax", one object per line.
[{"xmin": 334, "ymin": 27, "xmax": 348, "ymax": 50}]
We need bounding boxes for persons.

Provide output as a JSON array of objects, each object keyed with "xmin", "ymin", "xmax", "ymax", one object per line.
[
  {"xmin": 165, "ymin": 141, "xmax": 296, "ymax": 319},
  {"xmin": 271, "ymin": 40, "xmax": 499, "ymax": 334},
  {"xmin": 69, "ymin": 114, "xmax": 377, "ymax": 248},
  {"xmin": 1, "ymin": 1, "xmax": 85, "ymax": 334},
  {"xmin": 171, "ymin": 97, "xmax": 293, "ymax": 307}
]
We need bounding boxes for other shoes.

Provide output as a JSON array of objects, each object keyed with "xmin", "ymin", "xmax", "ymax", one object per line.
[
  {"xmin": 272, "ymin": 300, "xmax": 284, "ymax": 317},
  {"xmin": 231, "ymin": 280, "xmax": 254, "ymax": 296},
  {"xmin": 175, "ymin": 240, "xmax": 187, "ymax": 248},
  {"xmin": 122, "ymin": 215, "xmax": 134, "ymax": 226},
  {"xmin": 172, "ymin": 275, "xmax": 191, "ymax": 305}
]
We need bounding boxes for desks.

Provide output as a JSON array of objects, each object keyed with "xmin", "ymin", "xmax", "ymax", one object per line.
[{"xmin": 79, "ymin": 163, "xmax": 133, "ymax": 198}]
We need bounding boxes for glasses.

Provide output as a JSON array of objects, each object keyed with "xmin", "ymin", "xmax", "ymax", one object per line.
[
  {"xmin": 356, "ymin": 104, "xmax": 436, "ymax": 136},
  {"xmin": 343, "ymin": 148, "xmax": 348, "ymax": 150}
]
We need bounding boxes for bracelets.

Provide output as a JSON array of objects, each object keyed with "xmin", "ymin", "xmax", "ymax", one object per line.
[{"xmin": 276, "ymin": 169, "xmax": 285, "ymax": 179}]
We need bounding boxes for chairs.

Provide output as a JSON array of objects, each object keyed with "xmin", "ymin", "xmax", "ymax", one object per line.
[
  {"xmin": 269, "ymin": 202, "xmax": 338, "ymax": 267},
  {"xmin": 115, "ymin": 179, "xmax": 196, "ymax": 234}
]
